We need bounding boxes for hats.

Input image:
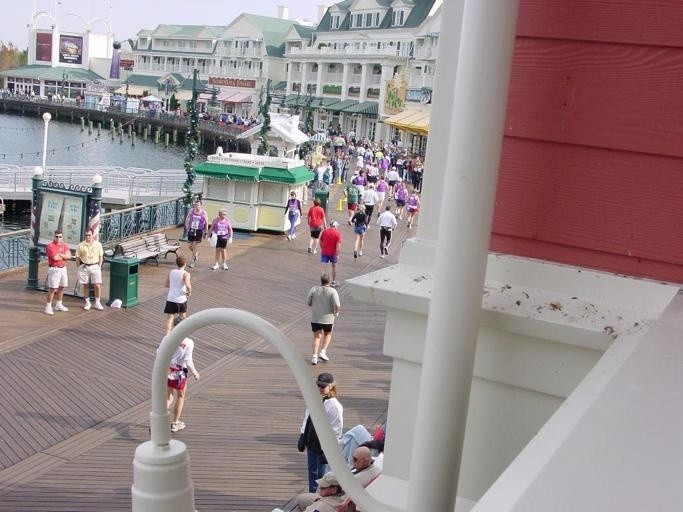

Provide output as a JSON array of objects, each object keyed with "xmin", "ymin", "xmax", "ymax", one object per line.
[
  {"xmin": 316, "ymin": 373, "xmax": 334, "ymax": 386},
  {"xmin": 330, "ymin": 220, "xmax": 339, "ymax": 227},
  {"xmin": 316, "ymin": 471, "xmax": 341, "ymax": 487}
]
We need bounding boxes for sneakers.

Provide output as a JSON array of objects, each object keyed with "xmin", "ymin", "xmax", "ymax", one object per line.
[
  {"xmin": 94, "ymin": 303, "xmax": 104, "ymax": 310},
  {"xmin": 318, "ymin": 353, "xmax": 329, "ymax": 360},
  {"xmin": 55, "ymin": 305, "xmax": 69, "ymax": 312},
  {"xmin": 211, "ymin": 264, "xmax": 219, "ymax": 270},
  {"xmin": 44, "ymin": 307, "xmax": 54, "ymax": 315},
  {"xmin": 331, "ymin": 281, "xmax": 339, "ymax": 286},
  {"xmin": 84, "ymin": 302, "xmax": 92, "ymax": 310},
  {"xmin": 354, "ymin": 250, "xmax": 362, "ymax": 258},
  {"xmin": 384, "ymin": 247, "xmax": 388, "ymax": 255},
  {"xmin": 222, "ymin": 264, "xmax": 228, "ymax": 270},
  {"xmin": 311, "ymin": 357, "xmax": 317, "ymax": 364},
  {"xmin": 189, "ymin": 252, "xmax": 200, "ymax": 268},
  {"xmin": 170, "ymin": 422, "xmax": 185, "ymax": 432},
  {"xmin": 308, "ymin": 247, "xmax": 317, "ymax": 254},
  {"xmin": 287, "ymin": 233, "xmax": 296, "ymax": 240}
]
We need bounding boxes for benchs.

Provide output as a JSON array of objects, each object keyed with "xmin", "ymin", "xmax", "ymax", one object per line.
[{"xmin": 100, "ymin": 233, "xmax": 183, "ymax": 270}]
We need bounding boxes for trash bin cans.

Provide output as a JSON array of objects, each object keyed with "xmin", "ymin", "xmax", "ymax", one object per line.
[
  {"xmin": 314, "ymin": 190, "xmax": 329, "ymax": 217},
  {"xmin": 105, "ymin": 257, "xmax": 141, "ymax": 308}
]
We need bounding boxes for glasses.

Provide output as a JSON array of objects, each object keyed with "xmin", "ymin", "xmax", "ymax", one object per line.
[
  {"xmin": 55, "ymin": 235, "xmax": 62, "ymax": 239},
  {"xmin": 84, "ymin": 234, "xmax": 91, "ymax": 236}
]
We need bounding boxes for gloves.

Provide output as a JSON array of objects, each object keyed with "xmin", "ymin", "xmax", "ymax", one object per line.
[{"xmin": 299, "ymin": 435, "xmax": 305, "ymax": 452}]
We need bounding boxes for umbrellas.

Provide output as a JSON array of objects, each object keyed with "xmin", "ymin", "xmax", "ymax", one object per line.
[{"xmin": 139, "ymin": 95, "xmax": 162, "ymax": 103}]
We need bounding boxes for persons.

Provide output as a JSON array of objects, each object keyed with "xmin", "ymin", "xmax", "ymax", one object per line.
[
  {"xmin": 297, "ymin": 372, "xmax": 387, "ymax": 512},
  {"xmin": 149, "ymin": 315, "xmax": 200, "ymax": 436},
  {"xmin": 75, "ymin": 227, "xmax": 104, "ymax": 310},
  {"xmin": 52, "ymin": 94, "xmax": 65, "ymax": 103},
  {"xmin": 284, "ymin": 115, "xmax": 424, "ymax": 286},
  {"xmin": 183, "ymin": 200, "xmax": 233, "ymax": 270},
  {"xmin": 161, "ymin": 103, "xmax": 258, "ymax": 127},
  {"xmin": 44, "ymin": 230, "xmax": 72, "ymax": 315},
  {"xmin": 0, "ymin": 89, "xmax": 35, "ymax": 100},
  {"xmin": 306, "ymin": 273, "xmax": 341, "ymax": 365},
  {"xmin": 164, "ymin": 256, "xmax": 191, "ymax": 337}
]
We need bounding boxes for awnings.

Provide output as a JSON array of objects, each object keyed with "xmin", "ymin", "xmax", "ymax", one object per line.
[
  {"xmin": 284, "ymin": 94, "xmax": 378, "ymax": 115},
  {"xmin": 384, "ymin": 110, "xmax": 428, "ymax": 135}
]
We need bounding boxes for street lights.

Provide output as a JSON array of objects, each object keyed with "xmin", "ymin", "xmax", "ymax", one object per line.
[
  {"xmin": 122, "ymin": 75, "xmax": 135, "ymax": 114},
  {"xmin": 60, "ymin": 70, "xmax": 70, "ymax": 105},
  {"xmin": 254, "ymin": 79, "xmax": 324, "ymax": 156},
  {"xmin": 82, "ymin": 173, "xmax": 104, "ymax": 301},
  {"xmin": 176, "ymin": 70, "xmax": 221, "ymax": 242},
  {"xmin": 23, "ymin": 166, "xmax": 43, "ymax": 291},
  {"xmin": 42, "ymin": 111, "xmax": 51, "ymax": 177}
]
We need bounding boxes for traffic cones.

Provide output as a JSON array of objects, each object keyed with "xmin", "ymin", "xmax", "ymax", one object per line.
[
  {"xmin": 335, "ymin": 199, "xmax": 343, "ymax": 211},
  {"xmin": 336, "ymin": 175, "xmax": 342, "ymax": 185},
  {"xmin": 340, "ymin": 189, "xmax": 347, "ymax": 201}
]
